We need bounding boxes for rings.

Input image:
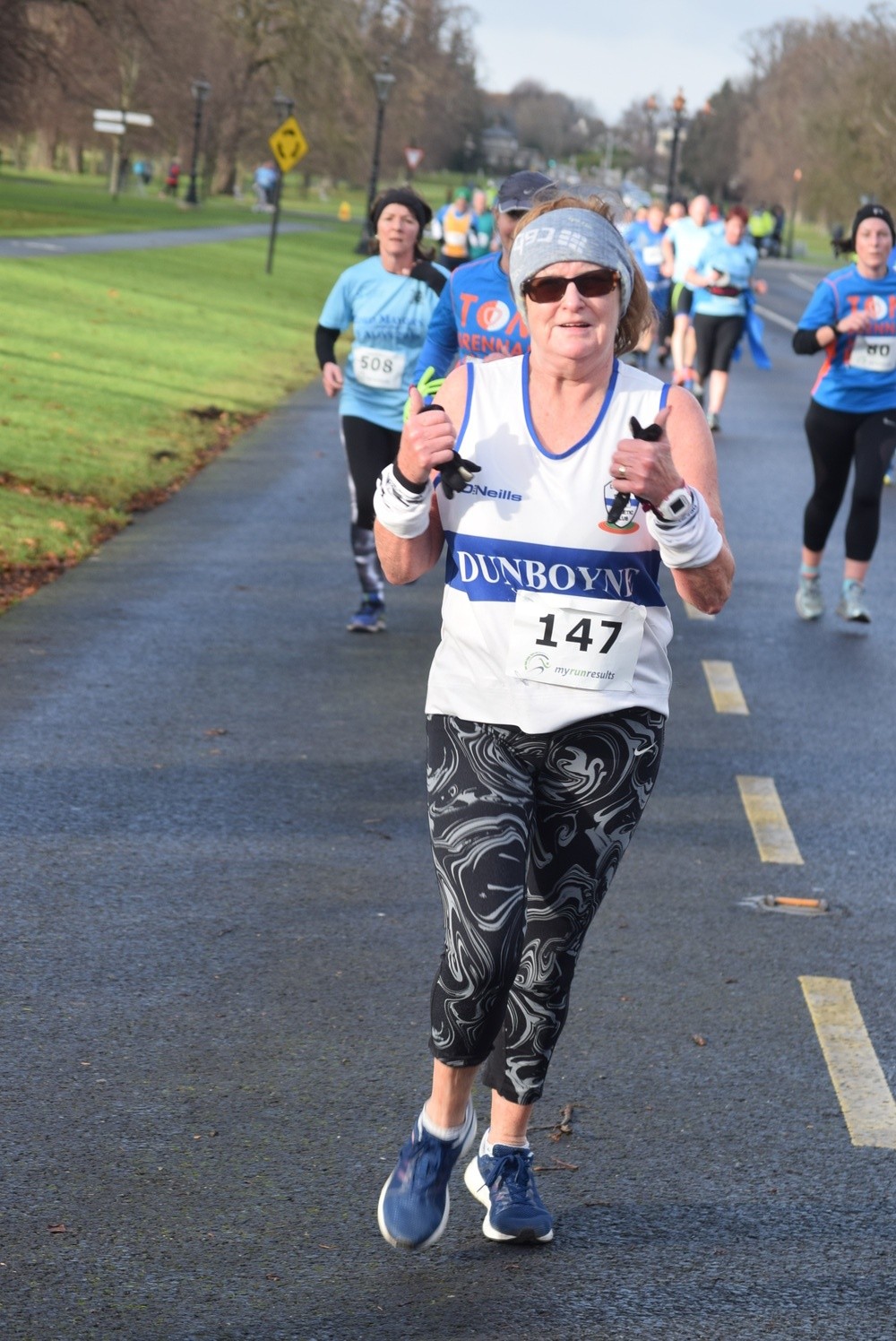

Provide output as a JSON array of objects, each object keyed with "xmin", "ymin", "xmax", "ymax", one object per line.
[{"xmin": 618, "ymin": 464, "xmax": 626, "ymax": 476}]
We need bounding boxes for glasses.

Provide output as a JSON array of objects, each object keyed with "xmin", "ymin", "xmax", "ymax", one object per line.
[{"xmin": 522, "ymin": 267, "xmax": 622, "ymax": 303}]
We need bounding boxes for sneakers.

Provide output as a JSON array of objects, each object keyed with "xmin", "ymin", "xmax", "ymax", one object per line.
[
  {"xmin": 465, "ymin": 1127, "xmax": 553, "ymax": 1243},
  {"xmin": 794, "ymin": 580, "xmax": 824, "ymax": 618},
  {"xmin": 378, "ymin": 1092, "xmax": 478, "ymax": 1253},
  {"xmin": 345, "ymin": 604, "xmax": 389, "ymax": 631},
  {"xmin": 834, "ymin": 596, "xmax": 871, "ymax": 622}
]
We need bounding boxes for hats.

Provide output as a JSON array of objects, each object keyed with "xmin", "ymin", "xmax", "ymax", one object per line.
[{"xmin": 497, "ymin": 172, "xmax": 557, "ymax": 213}]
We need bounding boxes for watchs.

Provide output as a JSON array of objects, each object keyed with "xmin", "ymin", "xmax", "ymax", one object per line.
[{"xmin": 654, "ymin": 487, "xmax": 695, "ymax": 520}]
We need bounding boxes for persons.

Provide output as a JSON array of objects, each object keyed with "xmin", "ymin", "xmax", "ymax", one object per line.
[
  {"xmin": 791, "ymin": 205, "xmax": 896, "ymax": 624},
  {"xmin": 312, "ymin": 190, "xmax": 449, "ymax": 633},
  {"xmin": 373, "ymin": 198, "xmax": 734, "ymax": 1253},
  {"xmin": 831, "ymin": 226, "xmax": 847, "ymax": 262},
  {"xmin": 251, "ymin": 155, "xmax": 280, "ymax": 212},
  {"xmin": 160, "ymin": 158, "xmax": 182, "ymax": 201},
  {"xmin": 133, "ymin": 156, "xmax": 153, "ymax": 196},
  {"xmin": 405, "ymin": 170, "xmax": 784, "ymax": 434}
]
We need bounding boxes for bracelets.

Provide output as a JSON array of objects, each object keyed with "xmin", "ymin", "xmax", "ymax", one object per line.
[
  {"xmin": 828, "ymin": 321, "xmax": 842, "ymax": 339},
  {"xmin": 394, "ymin": 456, "xmax": 428, "ymax": 493}
]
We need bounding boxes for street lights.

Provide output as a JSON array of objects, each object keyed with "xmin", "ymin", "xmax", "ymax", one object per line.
[
  {"xmin": 666, "ymin": 87, "xmax": 686, "ymax": 205},
  {"xmin": 264, "ymin": 95, "xmax": 302, "ymax": 273},
  {"xmin": 354, "ymin": 56, "xmax": 398, "ymax": 256},
  {"xmin": 181, "ymin": 70, "xmax": 211, "ymax": 204}
]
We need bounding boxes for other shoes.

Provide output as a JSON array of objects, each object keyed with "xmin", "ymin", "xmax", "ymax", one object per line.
[
  {"xmin": 708, "ymin": 415, "xmax": 719, "ymax": 431},
  {"xmin": 693, "ymin": 393, "xmax": 703, "ymax": 407}
]
